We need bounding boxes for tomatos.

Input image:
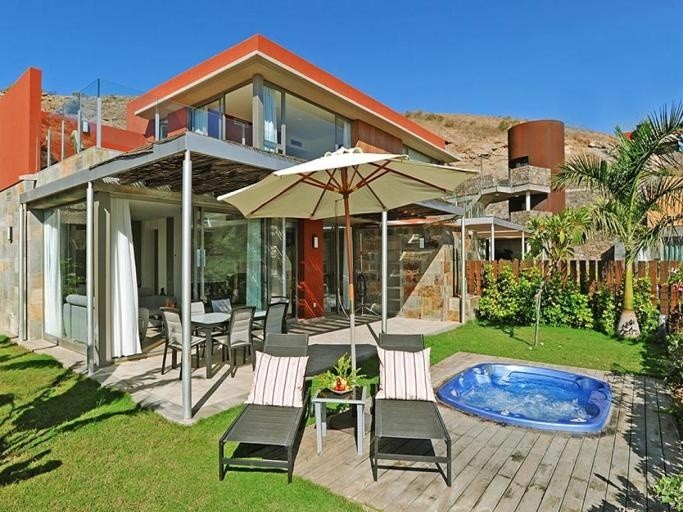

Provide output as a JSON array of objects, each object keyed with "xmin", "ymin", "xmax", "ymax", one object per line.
[{"xmin": 335, "ymin": 378, "xmax": 342, "ymax": 391}]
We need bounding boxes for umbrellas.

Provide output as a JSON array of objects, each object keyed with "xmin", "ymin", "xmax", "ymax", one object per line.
[{"xmin": 216, "ymin": 147, "xmax": 480, "ymax": 400}]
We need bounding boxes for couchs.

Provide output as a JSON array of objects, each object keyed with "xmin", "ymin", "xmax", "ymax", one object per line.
[
  {"xmin": 138, "ymin": 287, "xmax": 177, "ymax": 316},
  {"xmin": 62, "ymin": 294, "xmax": 149, "ymax": 346}
]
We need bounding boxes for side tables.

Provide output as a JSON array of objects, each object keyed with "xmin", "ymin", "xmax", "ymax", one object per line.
[{"xmin": 309, "ymin": 385, "xmax": 367, "ymax": 456}]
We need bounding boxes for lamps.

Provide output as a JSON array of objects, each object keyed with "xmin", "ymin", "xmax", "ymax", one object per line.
[
  {"xmin": 8, "ymin": 226, "xmax": 12, "ymax": 241},
  {"xmin": 419, "ymin": 237, "xmax": 425, "ymax": 249},
  {"xmin": 312, "ymin": 236, "xmax": 318, "ymax": 248}
]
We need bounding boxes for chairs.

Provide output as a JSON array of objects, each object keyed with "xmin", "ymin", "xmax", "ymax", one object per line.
[
  {"xmin": 157, "ymin": 295, "xmax": 289, "ymax": 380},
  {"xmin": 370, "ymin": 330, "xmax": 452, "ymax": 489},
  {"xmin": 216, "ymin": 332, "xmax": 310, "ymax": 484}
]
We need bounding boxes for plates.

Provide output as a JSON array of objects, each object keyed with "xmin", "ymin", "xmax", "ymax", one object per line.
[{"xmin": 326, "ymin": 386, "xmax": 354, "ymax": 395}]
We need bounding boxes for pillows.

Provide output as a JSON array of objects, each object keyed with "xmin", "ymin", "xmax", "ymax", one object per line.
[
  {"xmin": 241, "ymin": 347, "xmax": 308, "ymax": 408},
  {"xmin": 375, "ymin": 346, "xmax": 438, "ymax": 402}
]
